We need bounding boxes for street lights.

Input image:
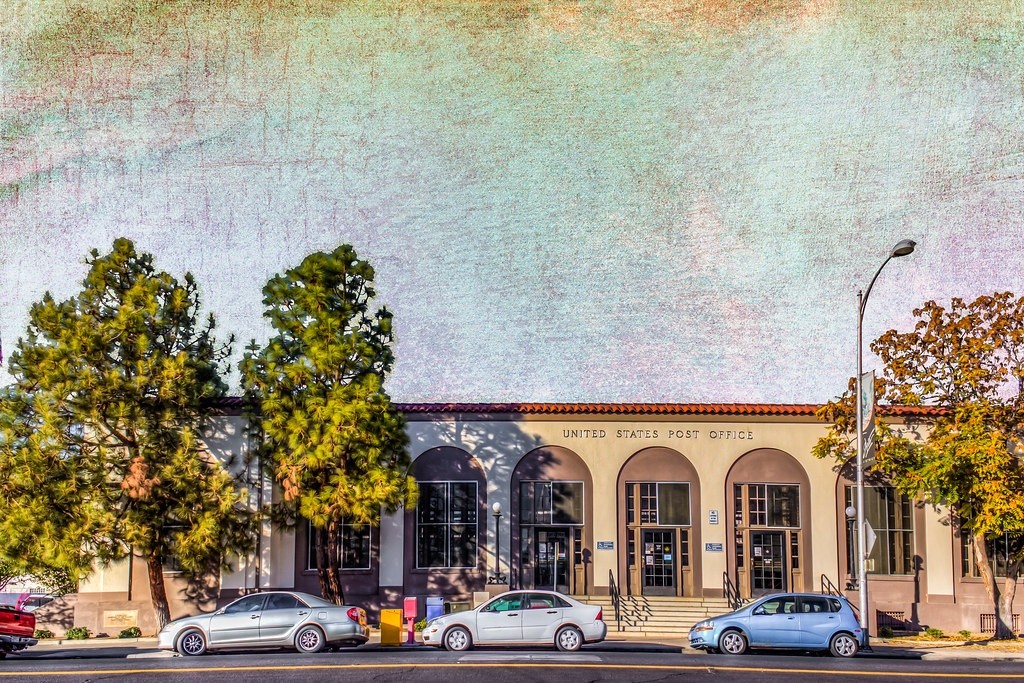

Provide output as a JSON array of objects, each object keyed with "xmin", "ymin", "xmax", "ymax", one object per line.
[
  {"xmin": 845, "ymin": 507, "xmax": 860, "ymax": 591},
  {"xmin": 855, "ymin": 238, "xmax": 918, "ymax": 646},
  {"xmin": 488, "ymin": 502, "xmax": 508, "ymax": 585}
]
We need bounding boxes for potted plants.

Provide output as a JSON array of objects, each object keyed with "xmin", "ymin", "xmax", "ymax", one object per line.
[
  {"xmin": 119, "ymin": 627, "xmax": 142, "ymax": 638},
  {"xmin": 34, "ymin": 629, "xmax": 54, "ymax": 639},
  {"xmin": 64, "ymin": 627, "xmax": 93, "ymax": 640}
]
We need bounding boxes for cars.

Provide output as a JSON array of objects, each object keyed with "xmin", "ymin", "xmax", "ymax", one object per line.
[
  {"xmin": 159, "ymin": 591, "xmax": 369, "ymax": 658},
  {"xmin": 686, "ymin": 591, "xmax": 864, "ymax": 657},
  {"xmin": 20, "ymin": 593, "xmax": 58, "ymax": 613},
  {"xmin": 1, "ymin": 605, "xmax": 38, "ymax": 659},
  {"xmin": 422, "ymin": 590, "xmax": 607, "ymax": 651}
]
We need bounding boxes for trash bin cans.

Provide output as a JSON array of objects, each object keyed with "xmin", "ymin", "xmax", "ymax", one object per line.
[
  {"xmin": 426, "ymin": 597, "xmax": 444, "ymax": 623},
  {"xmin": 444, "ymin": 603, "xmax": 470, "ymax": 614},
  {"xmin": 381, "ymin": 609, "xmax": 403, "ymax": 646}
]
